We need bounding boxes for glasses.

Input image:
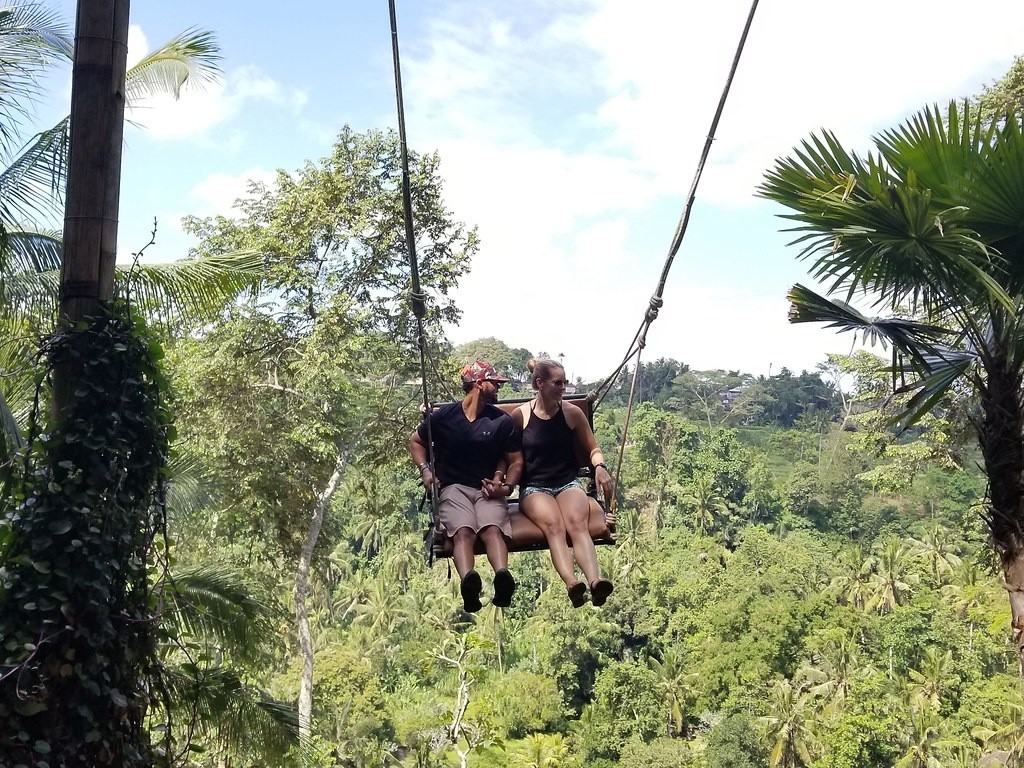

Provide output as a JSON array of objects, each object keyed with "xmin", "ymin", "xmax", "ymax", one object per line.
[{"xmin": 542, "ymin": 379, "xmax": 569, "ymax": 387}]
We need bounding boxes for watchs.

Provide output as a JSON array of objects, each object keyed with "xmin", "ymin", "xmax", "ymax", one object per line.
[
  {"xmin": 594, "ymin": 463, "xmax": 607, "ymax": 470},
  {"xmin": 503, "ymin": 484, "xmax": 513, "ymax": 496}
]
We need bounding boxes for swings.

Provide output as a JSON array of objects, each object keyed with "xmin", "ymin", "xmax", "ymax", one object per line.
[{"xmin": 388, "ymin": 0, "xmax": 762, "ymax": 558}]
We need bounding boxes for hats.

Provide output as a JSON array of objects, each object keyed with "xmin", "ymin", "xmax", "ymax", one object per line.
[{"xmin": 460, "ymin": 360, "xmax": 511, "ymax": 383}]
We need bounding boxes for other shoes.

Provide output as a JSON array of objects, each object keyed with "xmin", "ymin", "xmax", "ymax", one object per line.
[
  {"xmin": 492, "ymin": 568, "xmax": 515, "ymax": 607},
  {"xmin": 461, "ymin": 571, "xmax": 482, "ymax": 613},
  {"xmin": 568, "ymin": 581, "xmax": 586, "ymax": 608},
  {"xmin": 591, "ymin": 580, "xmax": 614, "ymax": 606}
]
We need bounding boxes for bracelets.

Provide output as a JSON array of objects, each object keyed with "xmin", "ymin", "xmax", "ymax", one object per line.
[
  {"xmin": 421, "ymin": 467, "xmax": 429, "ymax": 472},
  {"xmin": 419, "ymin": 462, "xmax": 427, "ymax": 469}
]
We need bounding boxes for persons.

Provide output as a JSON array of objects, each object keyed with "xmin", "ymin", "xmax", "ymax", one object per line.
[
  {"xmin": 408, "ymin": 358, "xmax": 524, "ymax": 613},
  {"xmin": 484, "ymin": 357, "xmax": 614, "ymax": 610}
]
